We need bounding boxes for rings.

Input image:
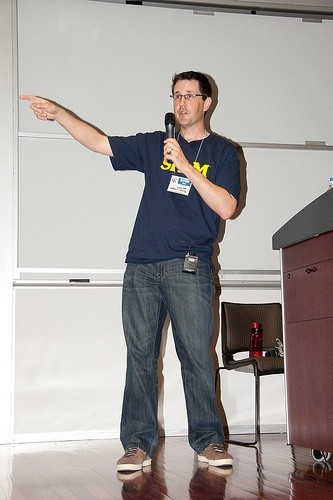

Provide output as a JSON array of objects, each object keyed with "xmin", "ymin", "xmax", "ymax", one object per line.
[{"xmin": 169, "ymin": 149, "xmax": 173, "ymax": 154}]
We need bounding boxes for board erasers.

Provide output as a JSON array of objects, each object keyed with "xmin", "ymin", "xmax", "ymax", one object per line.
[{"xmin": 304, "ymin": 140, "xmax": 326, "ymax": 146}]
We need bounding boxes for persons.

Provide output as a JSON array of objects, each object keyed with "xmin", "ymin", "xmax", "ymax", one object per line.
[{"xmin": 18, "ymin": 70, "xmax": 241, "ymax": 469}]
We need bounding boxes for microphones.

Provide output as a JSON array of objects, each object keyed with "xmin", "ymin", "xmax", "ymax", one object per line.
[{"xmin": 164, "ymin": 112, "xmax": 176, "ymax": 164}]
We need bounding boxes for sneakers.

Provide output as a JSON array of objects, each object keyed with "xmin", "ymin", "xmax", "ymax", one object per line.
[
  {"xmin": 115, "ymin": 447, "xmax": 152, "ymax": 470},
  {"xmin": 198, "ymin": 443, "xmax": 233, "ymax": 466}
]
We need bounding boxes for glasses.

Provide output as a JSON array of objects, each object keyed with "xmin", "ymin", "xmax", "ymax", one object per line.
[{"xmin": 170, "ymin": 94, "xmax": 206, "ymax": 101}]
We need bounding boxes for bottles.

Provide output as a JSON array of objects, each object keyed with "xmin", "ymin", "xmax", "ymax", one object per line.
[{"xmin": 249, "ymin": 323, "xmax": 263, "ymax": 359}]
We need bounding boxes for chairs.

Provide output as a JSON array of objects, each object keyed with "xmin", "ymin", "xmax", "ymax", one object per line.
[{"xmin": 214, "ymin": 302, "xmax": 284, "ymax": 445}]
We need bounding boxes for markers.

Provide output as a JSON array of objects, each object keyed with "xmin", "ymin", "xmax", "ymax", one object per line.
[{"xmin": 68, "ymin": 278, "xmax": 90, "ymax": 283}]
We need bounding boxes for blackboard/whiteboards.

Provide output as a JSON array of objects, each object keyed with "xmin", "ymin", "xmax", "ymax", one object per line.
[{"xmin": 13, "ymin": 1, "xmax": 333, "ymax": 286}]
